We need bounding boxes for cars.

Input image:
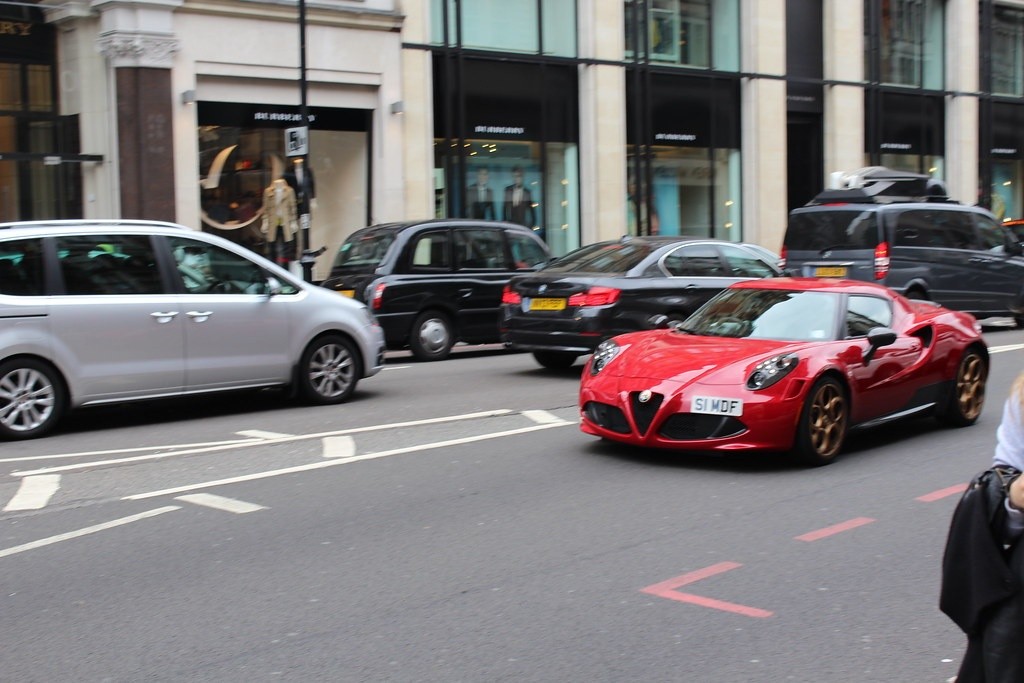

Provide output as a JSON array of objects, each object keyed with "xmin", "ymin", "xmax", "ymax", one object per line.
[
  {"xmin": 321, "ymin": 219, "xmax": 557, "ymax": 363},
  {"xmin": 500, "ymin": 236, "xmax": 785, "ymax": 371}
]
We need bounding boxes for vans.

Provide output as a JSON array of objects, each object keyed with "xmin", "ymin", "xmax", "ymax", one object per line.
[
  {"xmin": 779, "ymin": 202, "xmax": 1024, "ymax": 330},
  {"xmin": 0, "ymin": 217, "xmax": 386, "ymax": 441}
]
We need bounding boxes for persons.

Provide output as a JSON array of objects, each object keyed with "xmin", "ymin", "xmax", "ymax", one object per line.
[
  {"xmin": 625, "ymin": 172, "xmax": 658, "ymax": 237},
  {"xmin": 261, "ymin": 159, "xmax": 316, "ymax": 270},
  {"xmin": 940, "ymin": 374, "xmax": 1024, "ymax": 683},
  {"xmin": 464, "ymin": 166, "xmax": 537, "ymax": 230}
]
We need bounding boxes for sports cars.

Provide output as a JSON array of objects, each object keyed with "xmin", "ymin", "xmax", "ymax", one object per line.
[{"xmin": 577, "ymin": 279, "xmax": 991, "ymax": 466}]
